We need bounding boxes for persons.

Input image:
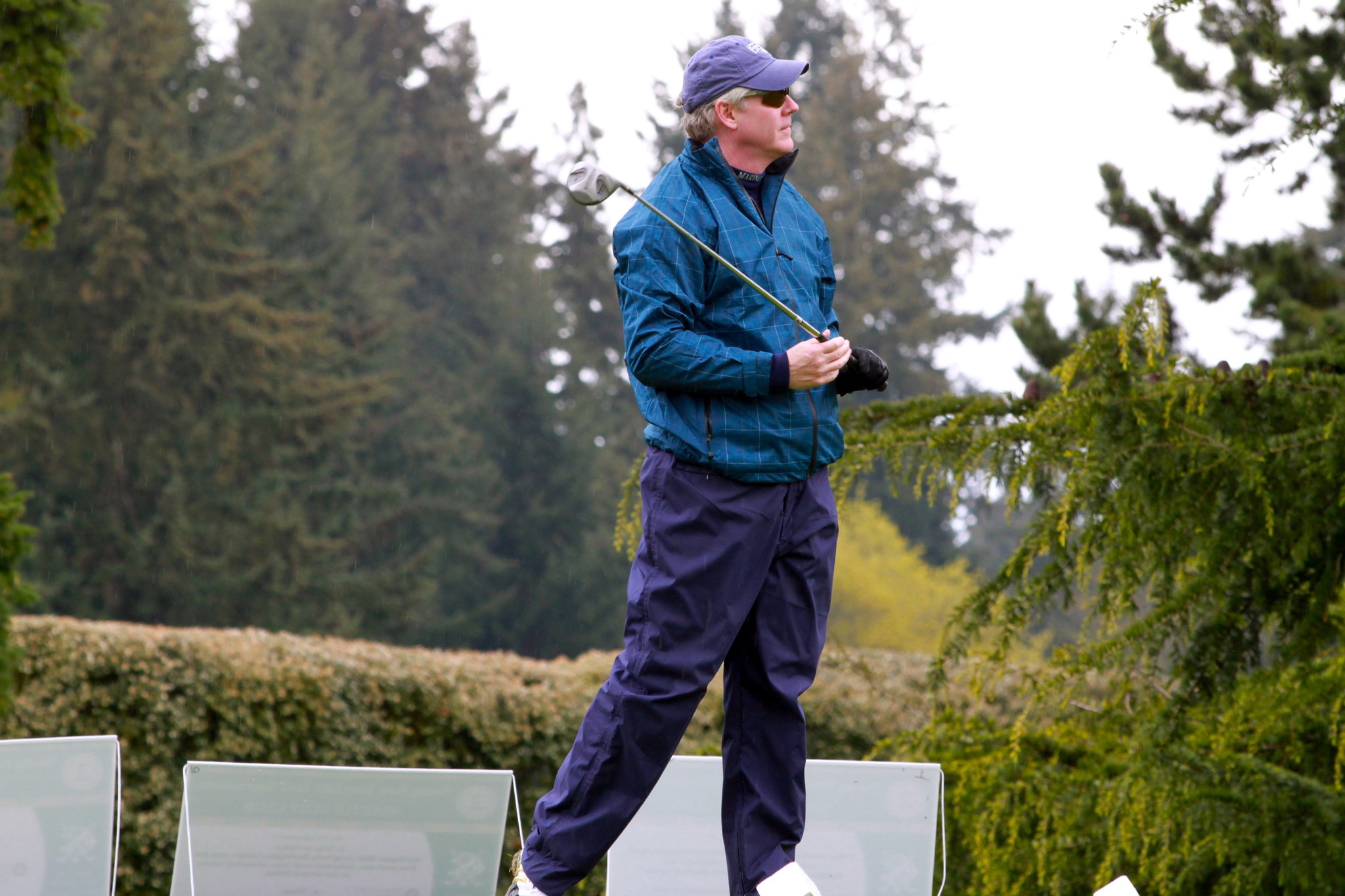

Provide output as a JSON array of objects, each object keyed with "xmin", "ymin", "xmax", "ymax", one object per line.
[{"xmin": 510, "ymin": 35, "xmax": 890, "ymax": 896}]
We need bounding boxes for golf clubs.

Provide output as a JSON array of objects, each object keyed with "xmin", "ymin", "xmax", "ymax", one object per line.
[{"xmin": 565, "ymin": 160, "xmax": 858, "ymax": 368}]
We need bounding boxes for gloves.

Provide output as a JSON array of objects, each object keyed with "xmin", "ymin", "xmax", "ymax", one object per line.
[{"xmin": 836, "ymin": 347, "xmax": 889, "ymax": 397}]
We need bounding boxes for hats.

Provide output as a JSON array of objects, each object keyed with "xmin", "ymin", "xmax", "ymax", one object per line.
[{"xmin": 683, "ymin": 35, "xmax": 810, "ymax": 113}]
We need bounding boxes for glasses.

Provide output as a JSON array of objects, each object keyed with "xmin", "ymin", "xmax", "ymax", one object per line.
[{"xmin": 742, "ymin": 87, "xmax": 791, "ymax": 110}]
